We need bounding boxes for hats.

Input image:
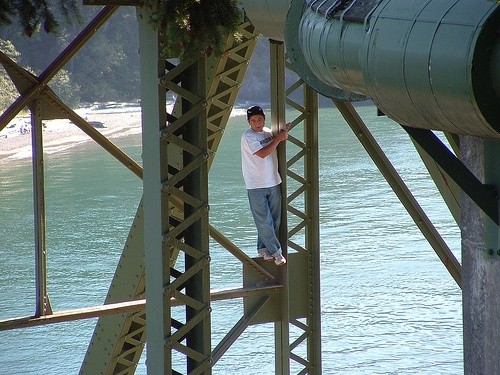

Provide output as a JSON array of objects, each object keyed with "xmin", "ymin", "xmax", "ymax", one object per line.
[{"xmin": 247, "ymin": 106, "xmax": 264, "ymax": 119}]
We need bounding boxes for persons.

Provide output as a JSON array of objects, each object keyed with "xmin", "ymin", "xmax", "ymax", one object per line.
[{"xmin": 240, "ymin": 104, "xmax": 292, "ymax": 265}]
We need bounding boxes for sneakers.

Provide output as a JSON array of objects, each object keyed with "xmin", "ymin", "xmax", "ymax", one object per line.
[
  {"xmin": 262, "ymin": 252, "xmax": 273, "ymax": 261},
  {"xmin": 274, "ymin": 254, "xmax": 286, "ymax": 267}
]
